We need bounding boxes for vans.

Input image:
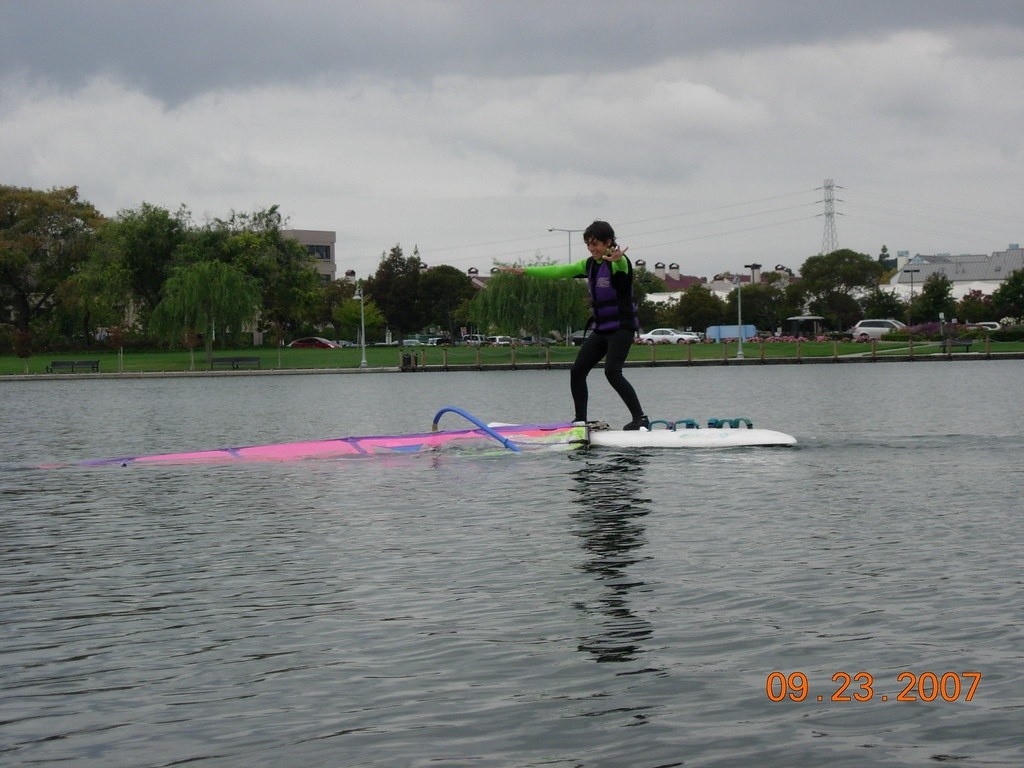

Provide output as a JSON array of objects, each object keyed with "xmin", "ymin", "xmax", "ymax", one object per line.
[{"xmin": 394, "ymin": 339, "xmax": 421, "ymax": 347}]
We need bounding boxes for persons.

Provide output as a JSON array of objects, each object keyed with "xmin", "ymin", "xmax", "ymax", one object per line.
[{"xmin": 498, "ymin": 220, "xmax": 648, "ymax": 431}]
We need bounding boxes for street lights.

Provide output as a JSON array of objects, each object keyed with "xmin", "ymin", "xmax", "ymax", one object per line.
[
  {"xmin": 548, "ymin": 227, "xmax": 586, "ymax": 266},
  {"xmin": 903, "ymin": 268, "xmax": 922, "ymax": 302}
]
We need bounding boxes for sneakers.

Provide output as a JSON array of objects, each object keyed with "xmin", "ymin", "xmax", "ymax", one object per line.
[{"xmin": 623, "ymin": 416, "xmax": 651, "ymax": 430}]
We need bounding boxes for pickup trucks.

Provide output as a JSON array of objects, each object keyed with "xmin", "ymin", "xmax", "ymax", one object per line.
[{"xmin": 455, "ymin": 334, "xmax": 490, "ymax": 347}]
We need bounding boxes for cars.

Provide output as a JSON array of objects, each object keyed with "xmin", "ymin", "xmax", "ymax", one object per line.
[
  {"xmin": 287, "ymin": 337, "xmax": 343, "ymax": 349},
  {"xmin": 966, "ymin": 321, "xmax": 1001, "ymax": 335},
  {"xmin": 487, "ymin": 336, "xmax": 511, "ymax": 346},
  {"xmin": 427, "ymin": 337, "xmax": 443, "ymax": 344},
  {"xmin": 523, "ymin": 336, "xmax": 539, "ymax": 344},
  {"xmin": 541, "ymin": 337, "xmax": 556, "ymax": 346},
  {"xmin": 568, "ymin": 328, "xmax": 595, "ymax": 346},
  {"xmin": 852, "ymin": 319, "xmax": 906, "ymax": 341},
  {"xmin": 636, "ymin": 327, "xmax": 701, "ymax": 346}
]
